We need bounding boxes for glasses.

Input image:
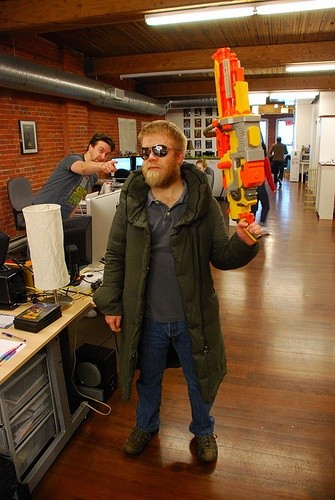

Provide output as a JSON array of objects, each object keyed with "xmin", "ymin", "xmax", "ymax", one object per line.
[{"xmin": 139, "ymin": 145, "xmax": 182, "ymax": 160}]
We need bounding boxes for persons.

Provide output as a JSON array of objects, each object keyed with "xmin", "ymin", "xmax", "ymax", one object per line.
[
  {"xmin": 196, "ymin": 159, "xmax": 212, "ymax": 188},
  {"xmin": 269, "ymin": 137, "xmax": 288, "ymax": 186},
  {"xmin": 32, "ymin": 133, "xmax": 119, "ymax": 218},
  {"xmin": 251, "ymin": 157, "xmax": 277, "ymax": 226},
  {"xmin": 93, "ymin": 120, "xmax": 263, "ymax": 464}
]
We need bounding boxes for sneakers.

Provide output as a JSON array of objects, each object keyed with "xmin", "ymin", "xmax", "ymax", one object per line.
[
  {"xmin": 123, "ymin": 426, "xmax": 159, "ymax": 456},
  {"xmin": 197, "ymin": 434, "xmax": 218, "ymax": 463}
]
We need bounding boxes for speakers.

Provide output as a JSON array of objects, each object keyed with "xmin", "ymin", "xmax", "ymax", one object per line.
[{"xmin": 73, "ymin": 343, "xmax": 119, "ymax": 402}]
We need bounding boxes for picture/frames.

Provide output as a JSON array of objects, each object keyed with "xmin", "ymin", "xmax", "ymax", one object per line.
[
  {"xmin": 19, "ymin": 120, "xmax": 39, "ymax": 155},
  {"xmin": 183, "ymin": 107, "xmax": 220, "ymax": 157}
]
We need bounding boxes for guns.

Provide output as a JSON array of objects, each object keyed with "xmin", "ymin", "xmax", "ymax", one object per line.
[{"xmin": 204, "ymin": 46, "xmax": 278, "ymax": 244}]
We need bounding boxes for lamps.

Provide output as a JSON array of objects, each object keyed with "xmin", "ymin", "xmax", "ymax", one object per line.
[
  {"xmin": 248, "ymin": 89, "xmax": 320, "ymax": 98},
  {"xmin": 285, "ymin": 60, "xmax": 335, "ymax": 71},
  {"xmin": 144, "ymin": 0, "xmax": 335, "ymax": 26}
]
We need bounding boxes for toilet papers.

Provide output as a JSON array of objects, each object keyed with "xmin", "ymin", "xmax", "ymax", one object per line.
[
  {"xmin": 22, "ymin": 203, "xmax": 71, "ymax": 291},
  {"xmin": 101, "ymin": 182, "xmax": 111, "ymax": 193}
]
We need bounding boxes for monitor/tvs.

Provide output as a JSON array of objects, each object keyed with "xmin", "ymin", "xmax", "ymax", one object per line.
[
  {"xmin": 63, "ymin": 217, "xmax": 93, "ymax": 284},
  {"xmin": 87, "ymin": 189, "xmax": 122, "ymax": 268},
  {"xmin": 133, "ymin": 155, "xmax": 144, "ymax": 171},
  {"xmin": 110, "ymin": 157, "xmax": 132, "ymax": 177},
  {"xmin": 0, "ymin": 231, "xmax": 11, "ymax": 271}
]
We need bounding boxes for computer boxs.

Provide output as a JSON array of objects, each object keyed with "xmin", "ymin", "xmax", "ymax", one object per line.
[{"xmin": 0, "ymin": 268, "xmax": 28, "ymax": 310}]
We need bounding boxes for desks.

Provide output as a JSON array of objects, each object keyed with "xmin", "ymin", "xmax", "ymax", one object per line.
[
  {"xmin": 301, "ymin": 162, "xmax": 309, "ymax": 182},
  {"xmin": 0, "ymin": 264, "xmax": 107, "ymax": 379}
]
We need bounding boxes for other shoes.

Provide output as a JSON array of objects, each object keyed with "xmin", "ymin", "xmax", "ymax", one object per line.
[
  {"xmin": 258, "ymin": 226, "xmax": 271, "ymax": 235},
  {"xmin": 86, "ymin": 309, "xmax": 97, "ymax": 318},
  {"xmin": 278, "ymin": 178, "xmax": 282, "ymax": 185}
]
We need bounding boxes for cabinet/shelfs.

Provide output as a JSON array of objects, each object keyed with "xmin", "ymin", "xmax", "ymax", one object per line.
[{"xmin": 0, "ymin": 346, "xmax": 70, "ymax": 500}]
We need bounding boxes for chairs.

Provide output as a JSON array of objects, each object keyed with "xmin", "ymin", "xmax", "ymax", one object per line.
[
  {"xmin": 206, "ymin": 167, "xmax": 215, "ymax": 191},
  {"xmin": 8, "ymin": 177, "xmax": 83, "ymax": 283}
]
